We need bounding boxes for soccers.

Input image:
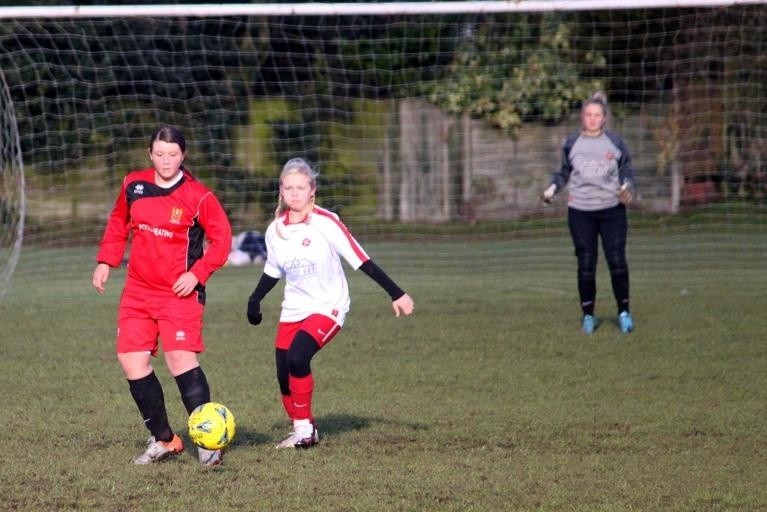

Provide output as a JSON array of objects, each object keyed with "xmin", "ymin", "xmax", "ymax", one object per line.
[{"xmin": 188, "ymin": 403, "xmax": 236, "ymax": 450}]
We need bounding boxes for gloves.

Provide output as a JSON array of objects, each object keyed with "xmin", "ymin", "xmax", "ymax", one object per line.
[{"xmin": 247, "ymin": 299, "xmax": 264, "ymax": 324}]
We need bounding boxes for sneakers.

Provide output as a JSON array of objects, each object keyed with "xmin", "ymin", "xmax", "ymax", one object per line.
[
  {"xmin": 581, "ymin": 316, "xmax": 598, "ymax": 334},
  {"xmin": 197, "ymin": 446, "xmax": 220, "ymax": 468},
  {"xmin": 619, "ymin": 313, "xmax": 633, "ymax": 334},
  {"xmin": 135, "ymin": 434, "xmax": 183, "ymax": 465},
  {"xmin": 277, "ymin": 426, "xmax": 320, "ymax": 448}
]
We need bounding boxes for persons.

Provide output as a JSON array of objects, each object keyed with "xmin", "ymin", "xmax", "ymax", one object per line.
[
  {"xmin": 541, "ymin": 90, "xmax": 636, "ymax": 336},
  {"xmin": 91, "ymin": 125, "xmax": 230, "ymax": 468},
  {"xmin": 246, "ymin": 157, "xmax": 414, "ymax": 448}
]
